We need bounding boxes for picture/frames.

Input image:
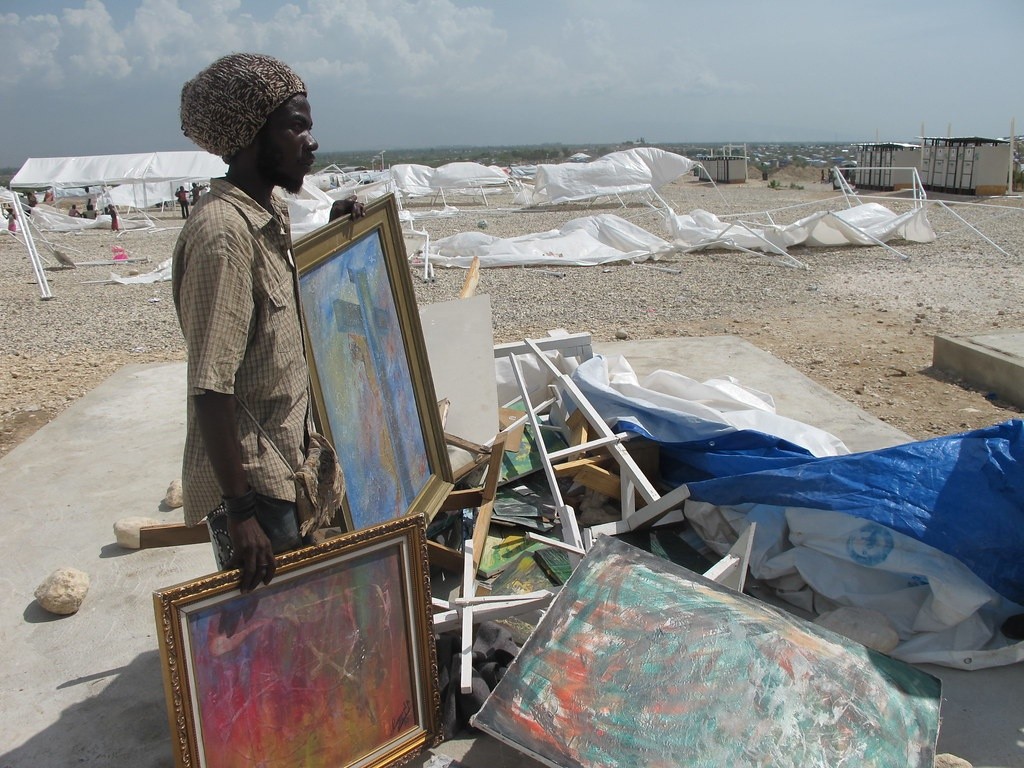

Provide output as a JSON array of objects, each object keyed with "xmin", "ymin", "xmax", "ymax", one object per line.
[
  {"xmin": 296, "ymin": 189, "xmax": 456, "ymax": 536},
  {"xmin": 154, "ymin": 511, "xmax": 447, "ymax": 768}
]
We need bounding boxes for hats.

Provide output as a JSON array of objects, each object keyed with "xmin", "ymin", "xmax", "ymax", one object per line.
[{"xmin": 181, "ymin": 53, "xmax": 307, "ymax": 163}]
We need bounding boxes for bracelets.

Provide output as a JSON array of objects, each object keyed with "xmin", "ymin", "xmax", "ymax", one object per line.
[{"xmin": 223, "ymin": 489, "xmax": 256, "ymax": 518}]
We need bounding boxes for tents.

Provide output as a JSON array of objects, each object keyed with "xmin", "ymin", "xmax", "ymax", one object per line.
[{"xmin": 9, "ymin": 150, "xmax": 230, "ymax": 227}]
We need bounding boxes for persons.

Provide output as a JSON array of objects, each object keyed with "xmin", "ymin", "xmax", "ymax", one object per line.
[
  {"xmin": 19, "ymin": 192, "xmax": 29, "ymax": 211},
  {"xmin": 85, "ymin": 187, "xmax": 97, "ymax": 217},
  {"xmin": 108, "ymin": 204, "xmax": 119, "ymax": 231},
  {"xmin": 172, "ymin": 53, "xmax": 367, "ymax": 593},
  {"xmin": 190, "ymin": 183, "xmax": 204, "ymax": 205},
  {"xmin": 828, "ymin": 169, "xmax": 832, "ymax": 184},
  {"xmin": 6, "ymin": 207, "xmax": 16, "ymax": 232},
  {"xmin": 176, "ymin": 186, "xmax": 189, "ymax": 218},
  {"xmin": 821, "ymin": 169, "xmax": 824, "ymax": 179},
  {"xmin": 26, "ymin": 192, "xmax": 37, "ymax": 207},
  {"xmin": 101, "ymin": 207, "xmax": 109, "ymax": 215},
  {"xmin": 69, "ymin": 205, "xmax": 82, "ymax": 217},
  {"xmin": 44, "ymin": 193, "xmax": 53, "ymax": 202}
]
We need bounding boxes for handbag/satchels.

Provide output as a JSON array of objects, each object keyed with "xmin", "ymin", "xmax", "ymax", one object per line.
[{"xmin": 295, "ymin": 433, "xmax": 346, "ymax": 538}]
